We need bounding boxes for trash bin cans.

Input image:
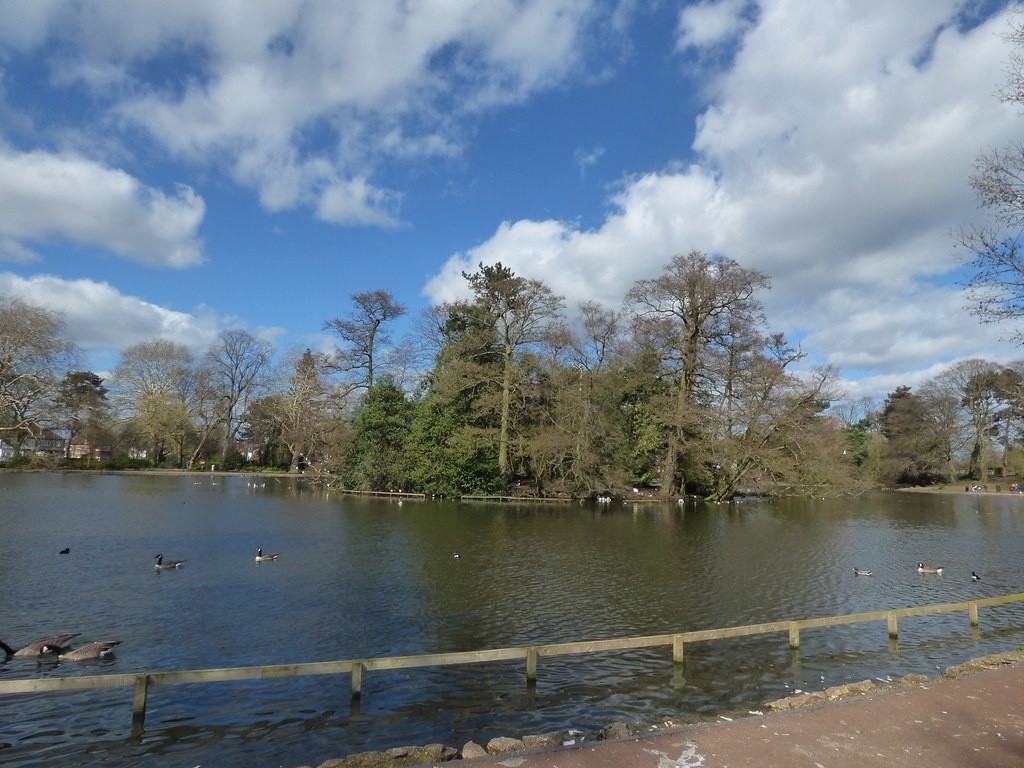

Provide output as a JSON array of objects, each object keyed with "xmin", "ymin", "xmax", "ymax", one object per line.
[
  {"xmin": 211, "ymin": 464, "xmax": 216, "ymax": 472},
  {"xmin": 996, "ymin": 485, "xmax": 1000, "ymax": 493}
]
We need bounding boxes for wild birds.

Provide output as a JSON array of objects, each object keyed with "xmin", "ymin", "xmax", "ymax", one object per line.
[
  {"xmin": 971, "ymin": 572, "xmax": 981, "ymax": 581},
  {"xmin": 851, "ymin": 566, "xmax": 873, "ymax": 576},
  {"xmin": 917, "ymin": 562, "xmax": 945, "ymax": 572},
  {"xmin": 153, "ymin": 554, "xmax": 187, "ymax": 569},
  {"xmin": 247, "ymin": 482, "xmax": 292, "ymax": 490},
  {"xmin": 0, "ymin": 633, "xmax": 125, "ymax": 662},
  {"xmin": 59, "ymin": 548, "xmax": 70, "ymax": 554},
  {"xmin": 452, "ymin": 551, "xmax": 462, "ymax": 559},
  {"xmin": 255, "ymin": 547, "xmax": 278, "ymax": 562}
]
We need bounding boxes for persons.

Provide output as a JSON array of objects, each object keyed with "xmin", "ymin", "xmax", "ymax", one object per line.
[{"xmin": 1009, "ymin": 483, "xmax": 1022, "ymax": 494}]
